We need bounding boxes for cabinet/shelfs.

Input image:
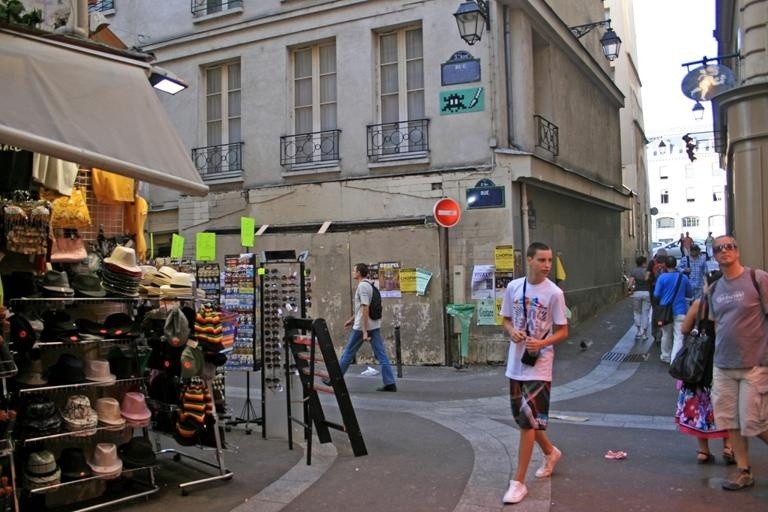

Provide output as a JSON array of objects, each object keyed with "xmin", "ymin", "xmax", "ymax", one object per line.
[
  {"xmin": 141, "ymin": 280, "xmax": 232, "ymax": 496},
  {"xmin": 1, "ymin": 305, "xmax": 21, "ymax": 511},
  {"xmin": 6, "ymin": 290, "xmax": 161, "ymax": 512}
]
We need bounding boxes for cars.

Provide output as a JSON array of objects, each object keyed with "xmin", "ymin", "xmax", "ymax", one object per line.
[
  {"xmin": 653, "ymin": 237, "xmax": 709, "ymax": 262},
  {"xmin": 651, "ymin": 241, "xmax": 668, "ymax": 255}
]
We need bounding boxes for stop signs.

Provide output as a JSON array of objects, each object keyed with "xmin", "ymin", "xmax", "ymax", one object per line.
[{"xmin": 431, "ymin": 196, "xmax": 461, "ymax": 228}]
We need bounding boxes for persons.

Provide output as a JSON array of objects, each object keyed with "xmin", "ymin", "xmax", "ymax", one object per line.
[
  {"xmin": 500, "ymin": 242, "xmax": 569, "ymax": 506},
  {"xmin": 628, "ymin": 232, "xmax": 714, "ymax": 367},
  {"xmin": 707, "ymin": 235, "xmax": 768, "ymax": 491},
  {"xmin": 323, "ymin": 263, "xmax": 397, "ymax": 392},
  {"xmin": 673, "ymin": 270, "xmax": 734, "ymax": 465}
]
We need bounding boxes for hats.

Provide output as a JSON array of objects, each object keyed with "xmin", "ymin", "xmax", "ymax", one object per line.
[
  {"xmin": 143, "ymin": 307, "xmax": 226, "ymax": 444},
  {"xmin": 10, "ymin": 245, "xmax": 216, "ymax": 302},
  {"xmin": 16, "ymin": 353, "xmax": 136, "ymax": 385},
  {"xmin": 22, "ymin": 435, "xmax": 156, "ymax": 494},
  {"xmin": 8, "ymin": 309, "xmax": 139, "ymax": 349},
  {"xmin": 21, "ymin": 392, "xmax": 152, "ymax": 436}
]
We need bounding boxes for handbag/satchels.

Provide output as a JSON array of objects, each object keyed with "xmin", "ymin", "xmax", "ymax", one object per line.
[
  {"xmin": 50, "ymin": 236, "xmax": 88, "ymax": 263},
  {"xmin": 2, "ymin": 189, "xmax": 91, "ymax": 228},
  {"xmin": 653, "ymin": 303, "xmax": 673, "ymax": 326},
  {"xmin": 669, "ymin": 328, "xmax": 716, "ymax": 385}
]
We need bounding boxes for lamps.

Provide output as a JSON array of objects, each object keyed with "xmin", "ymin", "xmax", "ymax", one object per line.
[
  {"xmin": 148, "ymin": 67, "xmax": 188, "ymax": 97},
  {"xmin": 682, "ymin": 129, "xmax": 728, "ymax": 163},
  {"xmin": 452, "ymin": 0, "xmax": 492, "ymax": 47},
  {"xmin": 567, "ymin": 18, "xmax": 623, "ymax": 63},
  {"xmin": 643, "ymin": 135, "xmax": 667, "ymax": 156},
  {"xmin": 680, "ymin": 53, "xmax": 741, "ymax": 121}
]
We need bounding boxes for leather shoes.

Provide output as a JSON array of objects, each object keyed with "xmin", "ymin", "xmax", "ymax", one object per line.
[
  {"xmin": 377, "ymin": 384, "xmax": 397, "ymax": 392},
  {"xmin": 323, "ymin": 378, "xmax": 332, "ymax": 386}
]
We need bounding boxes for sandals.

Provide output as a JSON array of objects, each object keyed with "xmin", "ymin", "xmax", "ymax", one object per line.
[
  {"xmin": 697, "ymin": 448, "xmax": 715, "ymax": 464},
  {"xmin": 723, "ymin": 446, "xmax": 736, "ymax": 464}
]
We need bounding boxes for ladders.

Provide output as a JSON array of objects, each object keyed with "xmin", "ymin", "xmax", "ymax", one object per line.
[{"xmin": 283, "ymin": 316, "xmax": 368, "ymax": 465}]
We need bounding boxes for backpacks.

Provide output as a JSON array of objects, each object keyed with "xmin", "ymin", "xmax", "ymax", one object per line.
[{"xmin": 359, "ymin": 280, "xmax": 382, "ymax": 319}]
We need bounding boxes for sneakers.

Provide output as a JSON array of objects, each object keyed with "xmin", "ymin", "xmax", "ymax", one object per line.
[
  {"xmin": 637, "ymin": 330, "xmax": 648, "ymax": 340},
  {"xmin": 660, "ymin": 354, "xmax": 670, "ymax": 363},
  {"xmin": 722, "ymin": 466, "xmax": 754, "ymax": 489},
  {"xmin": 502, "ymin": 480, "xmax": 528, "ymax": 503},
  {"xmin": 535, "ymin": 446, "xmax": 562, "ymax": 478}
]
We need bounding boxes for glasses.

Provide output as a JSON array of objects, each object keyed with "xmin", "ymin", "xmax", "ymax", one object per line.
[
  {"xmin": 304, "ymin": 268, "xmax": 312, "ymax": 307},
  {"xmin": 265, "ymin": 378, "xmax": 283, "ymax": 390},
  {"xmin": 713, "ymin": 244, "xmax": 737, "ymax": 254},
  {"xmin": 263, "ymin": 268, "xmax": 296, "ymax": 369}
]
